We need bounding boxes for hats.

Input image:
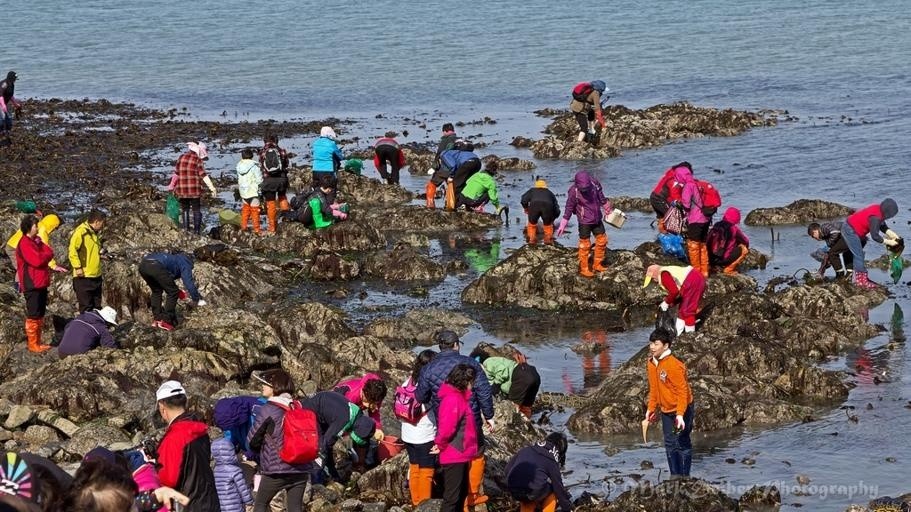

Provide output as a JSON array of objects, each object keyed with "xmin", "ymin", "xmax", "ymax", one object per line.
[
  {"xmin": 643, "ymin": 276, "xmax": 652, "ymax": 288},
  {"xmin": 534, "ymin": 180, "xmax": 548, "ymax": 188},
  {"xmin": 154, "ymin": 379, "xmax": 187, "ymax": 412},
  {"xmin": 673, "ymin": 181, "xmax": 679, "ymax": 188},
  {"xmin": 93, "ymin": 305, "xmax": 119, "ymax": 326},
  {"xmin": 437, "ymin": 330, "xmax": 459, "ymax": 345},
  {"xmin": 186, "ymin": 140, "xmax": 209, "ymax": 161}
]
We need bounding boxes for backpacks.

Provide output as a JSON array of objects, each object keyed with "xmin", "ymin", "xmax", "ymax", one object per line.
[
  {"xmin": 267, "ymin": 399, "xmax": 319, "ymax": 464},
  {"xmin": 709, "ymin": 221, "xmax": 730, "ymax": 259},
  {"xmin": 394, "ymin": 377, "xmax": 433, "ymax": 426},
  {"xmin": 453, "ymin": 138, "xmax": 474, "ymax": 152},
  {"xmin": 691, "ymin": 180, "xmax": 721, "ymax": 216},
  {"xmin": 290, "ymin": 187, "xmax": 314, "ymax": 211},
  {"xmin": 262, "ymin": 146, "xmax": 284, "ymax": 174},
  {"xmin": 571, "ymin": 82, "xmax": 592, "ymax": 102}
]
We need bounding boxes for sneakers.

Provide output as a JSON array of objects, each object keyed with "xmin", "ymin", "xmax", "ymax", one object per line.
[{"xmin": 158, "ymin": 320, "xmax": 176, "ymax": 331}]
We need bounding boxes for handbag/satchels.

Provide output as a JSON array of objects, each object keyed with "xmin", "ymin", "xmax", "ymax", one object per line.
[{"xmin": 661, "ymin": 200, "xmax": 683, "ymax": 235}]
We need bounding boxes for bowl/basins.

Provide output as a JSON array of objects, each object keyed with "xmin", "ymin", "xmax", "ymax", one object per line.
[{"xmin": 603, "ymin": 207, "xmax": 627, "ymax": 229}]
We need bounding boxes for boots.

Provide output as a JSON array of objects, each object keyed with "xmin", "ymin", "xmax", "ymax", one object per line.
[
  {"xmin": 278, "ymin": 195, "xmax": 290, "ymax": 211},
  {"xmin": 264, "ymin": 200, "xmax": 277, "ymax": 232},
  {"xmin": 193, "ymin": 212, "xmax": 203, "ymax": 235},
  {"xmin": 309, "ymin": 197, "xmax": 332, "ymax": 228},
  {"xmin": 856, "ymin": 271, "xmax": 876, "ymax": 287},
  {"xmin": 37, "ymin": 319, "xmax": 51, "ymax": 349},
  {"xmin": 578, "ymin": 237, "xmax": 594, "ymax": 276},
  {"xmin": 241, "ymin": 204, "xmax": 250, "ymax": 230},
  {"xmin": 527, "ymin": 221, "xmax": 539, "ymax": 243},
  {"xmin": 519, "ymin": 501, "xmax": 537, "ymax": 512},
  {"xmin": 723, "ymin": 244, "xmax": 749, "ymax": 274},
  {"xmin": 687, "ymin": 239, "xmax": 701, "ymax": 272},
  {"xmin": 587, "ymin": 120, "xmax": 596, "ymax": 134},
  {"xmin": 701, "ymin": 242, "xmax": 709, "ymax": 278},
  {"xmin": 675, "ymin": 317, "xmax": 685, "ymax": 336},
  {"xmin": 250, "ymin": 205, "xmax": 262, "ymax": 232},
  {"xmin": 183, "ymin": 210, "xmax": 190, "ymax": 231},
  {"xmin": 578, "ymin": 131, "xmax": 586, "ymax": 141},
  {"xmin": 24, "ymin": 318, "xmax": 44, "ymax": 352},
  {"xmin": 543, "ymin": 224, "xmax": 554, "ymax": 245},
  {"xmin": 466, "ymin": 454, "xmax": 489, "ymax": 506},
  {"xmin": 592, "ymin": 232, "xmax": 609, "ymax": 271},
  {"xmin": 408, "ymin": 463, "xmax": 420, "ymax": 506},
  {"xmin": 419, "ymin": 467, "xmax": 436, "ymax": 502},
  {"xmin": 541, "ymin": 492, "xmax": 557, "ymax": 512},
  {"xmin": 425, "ymin": 182, "xmax": 437, "ymax": 207},
  {"xmin": 685, "ymin": 325, "xmax": 696, "ymax": 332}
]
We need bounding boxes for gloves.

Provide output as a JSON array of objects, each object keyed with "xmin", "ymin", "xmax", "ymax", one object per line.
[
  {"xmin": 556, "ymin": 218, "xmax": 568, "ymax": 237},
  {"xmin": 646, "ymin": 410, "xmax": 657, "ymax": 423},
  {"xmin": 168, "ymin": 174, "xmax": 179, "ymax": 192},
  {"xmin": 332, "ymin": 209, "xmax": 348, "ymax": 220},
  {"xmin": 202, "ymin": 175, "xmax": 217, "ymax": 198},
  {"xmin": 885, "ymin": 228, "xmax": 900, "ymax": 240},
  {"xmin": 883, "ymin": 238, "xmax": 898, "ymax": 247},
  {"xmin": 658, "ymin": 301, "xmax": 670, "ymax": 312},
  {"xmin": 674, "ymin": 415, "xmax": 687, "ymax": 431},
  {"xmin": 603, "ymin": 200, "xmax": 613, "ymax": 215},
  {"xmin": 329, "ymin": 202, "xmax": 347, "ymax": 209}
]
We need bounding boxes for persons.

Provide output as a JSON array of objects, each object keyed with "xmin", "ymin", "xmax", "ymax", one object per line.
[
  {"xmin": 58, "ymin": 306, "xmax": 119, "ymax": 360},
  {"xmin": 650, "ymin": 161, "xmax": 693, "ymax": 222},
  {"xmin": 646, "ymin": 327, "xmax": 694, "ymax": 479},
  {"xmin": 310, "ymin": 126, "xmax": 344, "ymax": 203},
  {"xmin": 460, "ymin": 160, "xmax": 500, "ymax": 214},
  {"xmin": 469, "ymin": 349, "xmax": 541, "ymax": 418},
  {"xmin": 246, "ymin": 368, "xmax": 312, "ymax": 512},
  {"xmin": 644, "ymin": 264, "xmax": 705, "ymax": 337},
  {"xmin": 214, "ymin": 396, "xmax": 265, "ymax": 453},
  {"xmin": 0, "ymin": 446, "xmax": 190, "ymax": 512},
  {"xmin": 67, "ymin": 210, "xmax": 106, "ymax": 313},
  {"xmin": 393, "ymin": 349, "xmax": 439, "ymax": 507},
  {"xmin": 424, "ymin": 123, "xmax": 458, "ymax": 211},
  {"xmin": 840, "ymin": 198, "xmax": 900, "ymax": 289},
  {"xmin": 520, "ymin": 180, "xmax": 560, "ymax": 244},
  {"xmin": 212, "ymin": 437, "xmax": 254, "ymax": 512},
  {"xmin": 708, "ymin": 207, "xmax": 749, "ymax": 276},
  {"xmin": 557, "ymin": 172, "xmax": 611, "ymax": 276},
  {"xmin": 297, "ymin": 177, "xmax": 347, "ymax": 228},
  {"xmin": 569, "ymin": 80, "xmax": 606, "ymax": 142},
  {"xmin": 0, "ymin": 70, "xmax": 20, "ymax": 130},
  {"xmin": 168, "ymin": 141, "xmax": 216, "ymax": 235},
  {"xmin": 15, "ymin": 215, "xmax": 55, "ymax": 353},
  {"xmin": 435, "ymin": 150, "xmax": 482, "ymax": 211},
  {"xmin": 331, "ymin": 373, "xmax": 387, "ymax": 471},
  {"xmin": 260, "ymin": 129, "xmax": 291, "ymax": 236},
  {"xmin": 138, "ymin": 252, "xmax": 207, "ymax": 331},
  {"xmin": 504, "ymin": 432, "xmax": 570, "ymax": 512},
  {"xmin": 670, "ymin": 166, "xmax": 709, "ymax": 278},
  {"xmin": 373, "ymin": 138, "xmax": 405, "ymax": 185},
  {"xmin": 155, "ymin": 380, "xmax": 222, "ymax": 512},
  {"xmin": 807, "ymin": 221, "xmax": 867, "ymax": 280},
  {"xmin": 5, "ymin": 214, "xmax": 67, "ymax": 292},
  {"xmin": 415, "ymin": 330, "xmax": 495, "ymax": 511},
  {"xmin": 302, "ymin": 391, "xmax": 376, "ymax": 484},
  {"xmin": 429, "ymin": 364, "xmax": 479, "ymax": 512},
  {"xmin": 235, "ymin": 149, "xmax": 263, "ymax": 233}
]
